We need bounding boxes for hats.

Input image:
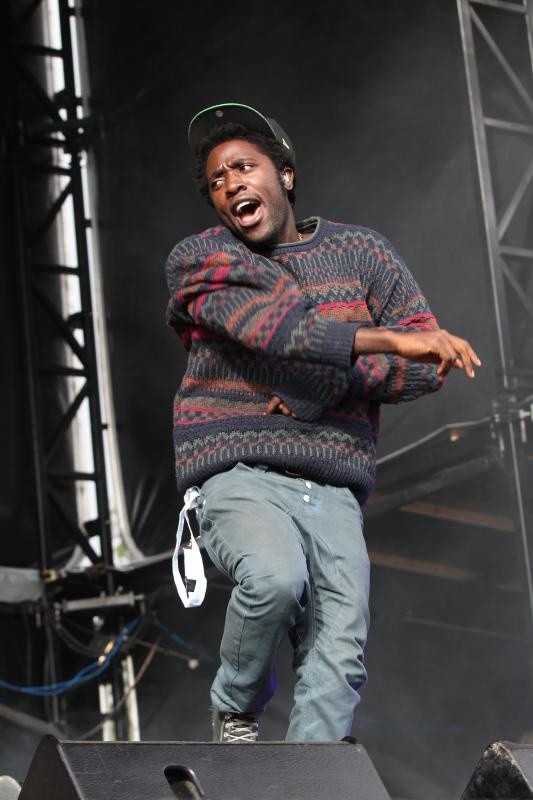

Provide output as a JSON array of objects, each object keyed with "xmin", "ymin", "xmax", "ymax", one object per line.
[{"xmin": 187, "ymin": 102, "xmax": 296, "ymax": 163}]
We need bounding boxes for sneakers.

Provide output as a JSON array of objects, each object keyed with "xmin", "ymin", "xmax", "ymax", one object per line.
[{"xmin": 210, "ymin": 707, "xmax": 261, "ymax": 744}]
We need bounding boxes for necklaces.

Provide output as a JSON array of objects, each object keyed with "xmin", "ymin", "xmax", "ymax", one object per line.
[{"xmin": 299, "ymin": 233, "xmax": 302, "ymax": 241}]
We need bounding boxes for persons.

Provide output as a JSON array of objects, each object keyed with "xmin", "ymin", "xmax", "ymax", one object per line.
[{"xmin": 165, "ymin": 104, "xmax": 484, "ymax": 742}]
